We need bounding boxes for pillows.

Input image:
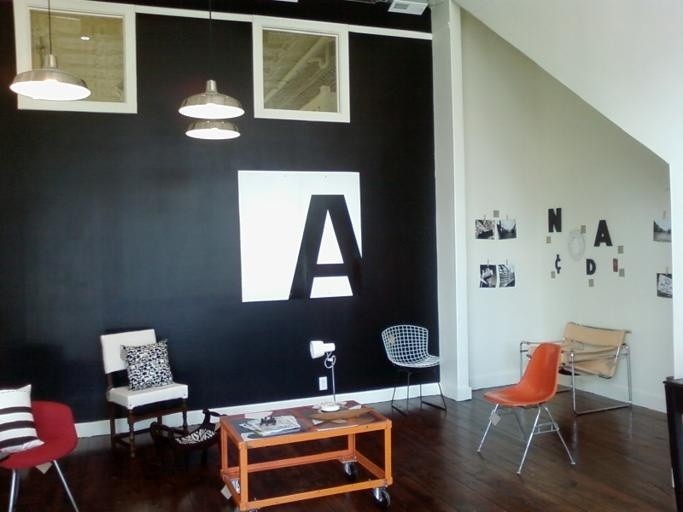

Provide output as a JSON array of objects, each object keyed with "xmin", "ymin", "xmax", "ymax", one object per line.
[
  {"xmin": 0, "ymin": 384, "xmax": 45, "ymax": 454},
  {"xmin": 120, "ymin": 338, "xmax": 174, "ymax": 391}
]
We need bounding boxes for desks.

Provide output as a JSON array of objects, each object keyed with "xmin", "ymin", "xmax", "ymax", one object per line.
[
  {"xmin": 662, "ymin": 378, "xmax": 682, "ymax": 512},
  {"xmin": 219, "ymin": 401, "xmax": 394, "ymax": 511}
]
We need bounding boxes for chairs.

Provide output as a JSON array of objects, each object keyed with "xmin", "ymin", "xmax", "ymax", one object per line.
[
  {"xmin": 101, "ymin": 329, "xmax": 188, "ymax": 459},
  {"xmin": 0, "ymin": 400, "xmax": 79, "ymax": 512},
  {"xmin": 519, "ymin": 321, "xmax": 633, "ymax": 416},
  {"xmin": 382, "ymin": 325, "xmax": 447, "ymax": 416},
  {"xmin": 476, "ymin": 343, "xmax": 575, "ymax": 474}
]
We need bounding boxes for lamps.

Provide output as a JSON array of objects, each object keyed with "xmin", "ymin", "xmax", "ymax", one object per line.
[
  {"xmin": 311, "ymin": 340, "xmax": 340, "ymax": 412},
  {"xmin": 179, "ymin": 0, "xmax": 246, "ymax": 121},
  {"xmin": 185, "ymin": 118, "xmax": 241, "ymax": 140},
  {"xmin": 10, "ymin": 0, "xmax": 91, "ymax": 101}
]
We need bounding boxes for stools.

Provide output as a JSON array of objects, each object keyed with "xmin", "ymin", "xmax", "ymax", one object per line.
[{"xmin": 151, "ymin": 408, "xmax": 228, "ymax": 486}]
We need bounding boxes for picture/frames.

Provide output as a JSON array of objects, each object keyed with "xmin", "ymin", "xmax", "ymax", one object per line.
[
  {"xmin": 252, "ymin": 15, "xmax": 351, "ymax": 123},
  {"xmin": 13, "ymin": 0, "xmax": 138, "ymax": 114}
]
